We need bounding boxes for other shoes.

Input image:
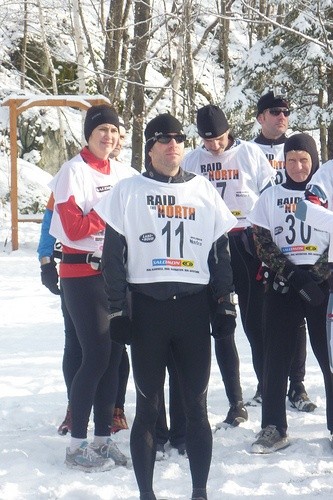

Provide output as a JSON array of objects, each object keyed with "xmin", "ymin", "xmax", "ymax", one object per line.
[
  {"xmin": 111, "ymin": 408, "xmax": 129, "ymax": 434},
  {"xmin": 178, "ymin": 443, "xmax": 189, "ymax": 459},
  {"xmin": 330, "ymin": 431, "xmax": 333, "ymax": 446},
  {"xmin": 155, "ymin": 443, "xmax": 165, "ymax": 461},
  {"xmin": 58, "ymin": 410, "xmax": 72, "ymax": 435}
]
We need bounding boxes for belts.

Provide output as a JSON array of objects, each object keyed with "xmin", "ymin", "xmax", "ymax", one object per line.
[{"xmin": 62, "ymin": 252, "xmax": 101, "ymax": 264}]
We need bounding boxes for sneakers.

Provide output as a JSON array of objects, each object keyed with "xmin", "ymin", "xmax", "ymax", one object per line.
[
  {"xmin": 244, "ymin": 397, "xmax": 262, "ymax": 407},
  {"xmin": 288, "ymin": 383, "xmax": 317, "ymax": 412},
  {"xmin": 215, "ymin": 402, "xmax": 248, "ymax": 428},
  {"xmin": 250, "ymin": 424, "xmax": 289, "ymax": 454},
  {"xmin": 64, "ymin": 441, "xmax": 115, "ymax": 473},
  {"xmin": 90, "ymin": 438, "xmax": 127, "ymax": 466}
]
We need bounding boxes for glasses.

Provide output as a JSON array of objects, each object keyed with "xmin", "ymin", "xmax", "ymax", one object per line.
[
  {"xmin": 266, "ymin": 107, "xmax": 290, "ymax": 116},
  {"xmin": 148, "ymin": 133, "xmax": 186, "ymax": 143}
]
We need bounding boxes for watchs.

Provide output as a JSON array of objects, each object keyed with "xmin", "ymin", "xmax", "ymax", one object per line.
[{"xmin": 219, "ymin": 291, "xmax": 237, "ymax": 305}]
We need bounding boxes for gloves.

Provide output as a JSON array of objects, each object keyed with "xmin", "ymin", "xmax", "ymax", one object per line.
[
  {"xmin": 286, "ymin": 269, "xmax": 325, "ymax": 306},
  {"xmin": 211, "ymin": 300, "xmax": 237, "ymax": 338},
  {"xmin": 40, "ymin": 261, "xmax": 60, "ymax": 295},
  {"xmin": 109, "ymin": 302, "xmax": 129, "ymax": 340}
]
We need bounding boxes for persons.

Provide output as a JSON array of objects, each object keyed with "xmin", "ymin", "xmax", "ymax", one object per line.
[
  {"xmin": 37, "ymin": 88, "xmax": 333, "ymax": 472},
  {"xmin": 92, "ymin": 115, "xmax": 237, "ymax": 500}
]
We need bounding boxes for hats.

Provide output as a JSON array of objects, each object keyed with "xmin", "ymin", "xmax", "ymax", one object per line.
[
  {"xmin": 257, "ymin": 91, "xmax": 289, "ymax": 114},
  {"xmin": 144, "ymin": 113, "xmax": 183, "ymax": 151},
  {"xmin": 84, "ymin": 104, "xmax": 119, "ymax": 144},
  {"xmin": 284, "ymin": 133, "xmax": 319, "ymax": 175},
  {"xmin": 197, "ymin": 104, "xmax": 230, "ymax": 140}
]
